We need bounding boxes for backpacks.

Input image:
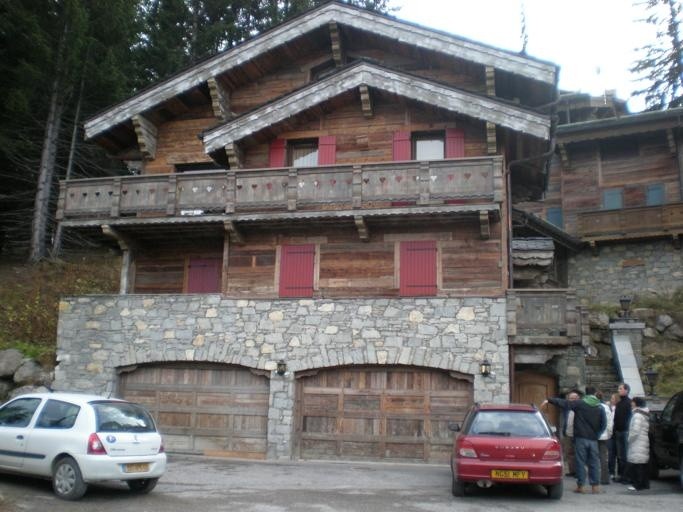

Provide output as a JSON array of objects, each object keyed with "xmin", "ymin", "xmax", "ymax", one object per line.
[{"xmin": 633, "ymin": 409, "xmax": 663, "ymax": 448}]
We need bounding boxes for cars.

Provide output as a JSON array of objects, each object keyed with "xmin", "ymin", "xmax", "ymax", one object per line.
[
  {"xmin": 0, "ymin": 392, "xmax": 167, "ymax": 500},
  {"xmin": 448, "ymin": 402, "xmax": 565, "ymax": 499},
  {"xmin": 648, "ymin": 390, "xmax": 683, "ymax": 490}
]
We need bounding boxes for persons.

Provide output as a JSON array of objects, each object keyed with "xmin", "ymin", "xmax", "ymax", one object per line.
[
  {"xmin": 605, "ymin": 393, "xmax": 621, "ymax": 479},
  {"xmin": 562, "ymin": 389, "xmax": 583, "ymax": 475},
  {"xmin": 540, "ymin": 382, "xmax": 606, "ymax": 495},
  {"xmin": 592, "ymin": 390, "xmax": 612, "ymax": 486},
  {"xmin": 612, "ymin": 383, "xmax": 632, "ymax": 483},
  {"xmin": 625, "ymin": 395, "xmax": 651, "ymax": 491}
]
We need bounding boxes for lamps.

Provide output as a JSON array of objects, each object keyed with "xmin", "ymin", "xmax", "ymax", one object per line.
[
  {"xmin": 275, "ymin": 359, "xmax": 287, "ymax": 377},
  {"xmin": 643, "ymin": 366, "xmax": 660, "ymax": 397},
  {"xmin": 479, "ymin": 359, "xmax": 492, "ymax": 376},
  {"xmin": 619, "ymin": 295, "xmax": 632, "ymax": 323}
]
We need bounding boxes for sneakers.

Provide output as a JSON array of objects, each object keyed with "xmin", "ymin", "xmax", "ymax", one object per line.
[
  {"xmin": 573, "ymin": 486, "xmax": 586, "ymax": 493},
  {"xmin": 609, "ymin": 474, "xmax": 621, "ymax": 480},
  {"xmin": 627, "ymin": 486, "xmax": 638, "ymax": 491},
  {"xmin": 565, "ymin": 472, "xmax": 577, "ymax": 478},
  {"xmin": 592, "ymin": 484, "xmax": 602, "ymax": 493},
  {"xmin": 601, "ymin": 480, "xmax": 611, "ymax": 485}
]
